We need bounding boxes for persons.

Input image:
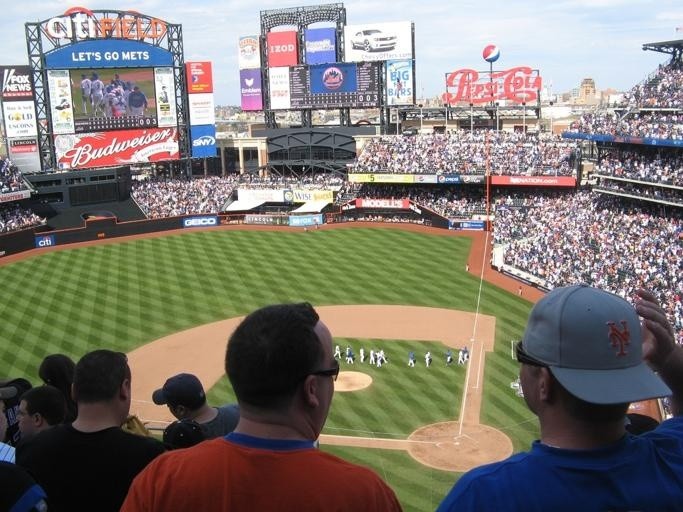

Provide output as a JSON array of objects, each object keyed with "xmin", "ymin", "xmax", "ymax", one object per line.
[
  {"xmin": 119, "ymin": 300, "xmax": 401, "ymax": 511},
  {"xmin": 81, "ymin": 72, "xmax": 147, "ymax": 117},
  {"xmin": 334, "ymin": 344, "xmax": 469, "ymax": 368},
  {"xmin": 1, "ymin": 202, "xmax": 48, "ymax": 233},
  {"xmin": 0, "ymin": 350, "xmax": 241, "ymax": 512},
  {"xmin": 130, "ymin": 173, "xmax": 348, "ymax": 224},
  {"xmin": 347, "ymin": 54, "xmax": 683, "ymax": 342},
  {"xmin": 435, "ymin": 282, "xmax": 683, "ymax": 512},
  {"xmin": 1, "ymin": 158, "xmax": 24, "ymax": 192}
]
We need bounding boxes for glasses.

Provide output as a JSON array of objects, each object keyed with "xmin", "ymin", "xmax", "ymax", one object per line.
[
  {"xmin": 515, "ymin": 339, "xmax": 548, "ymax": 367},
  {"xmin": 310, "ymin": 360, "xmax": 340, "ymax": 381}
]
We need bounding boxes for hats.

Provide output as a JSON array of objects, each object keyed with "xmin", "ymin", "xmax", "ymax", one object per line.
[
  {"xmin": 0, "ymin": 386, "xmax": 18, "ymax": 399},
  {"xmin": 153, "ymin": 374, "xmax": 204, "ymax": 405},
  {"xmin": 521, "ymin": 282, "xmax": 674, "ymax": 405},
  {"xmin": 39, "ymin": 355, "xmax": 74, "ymax": 383},
  {"xmin": 163, "ymin": 419, "xmax": 205, "ymax": 450}
]
